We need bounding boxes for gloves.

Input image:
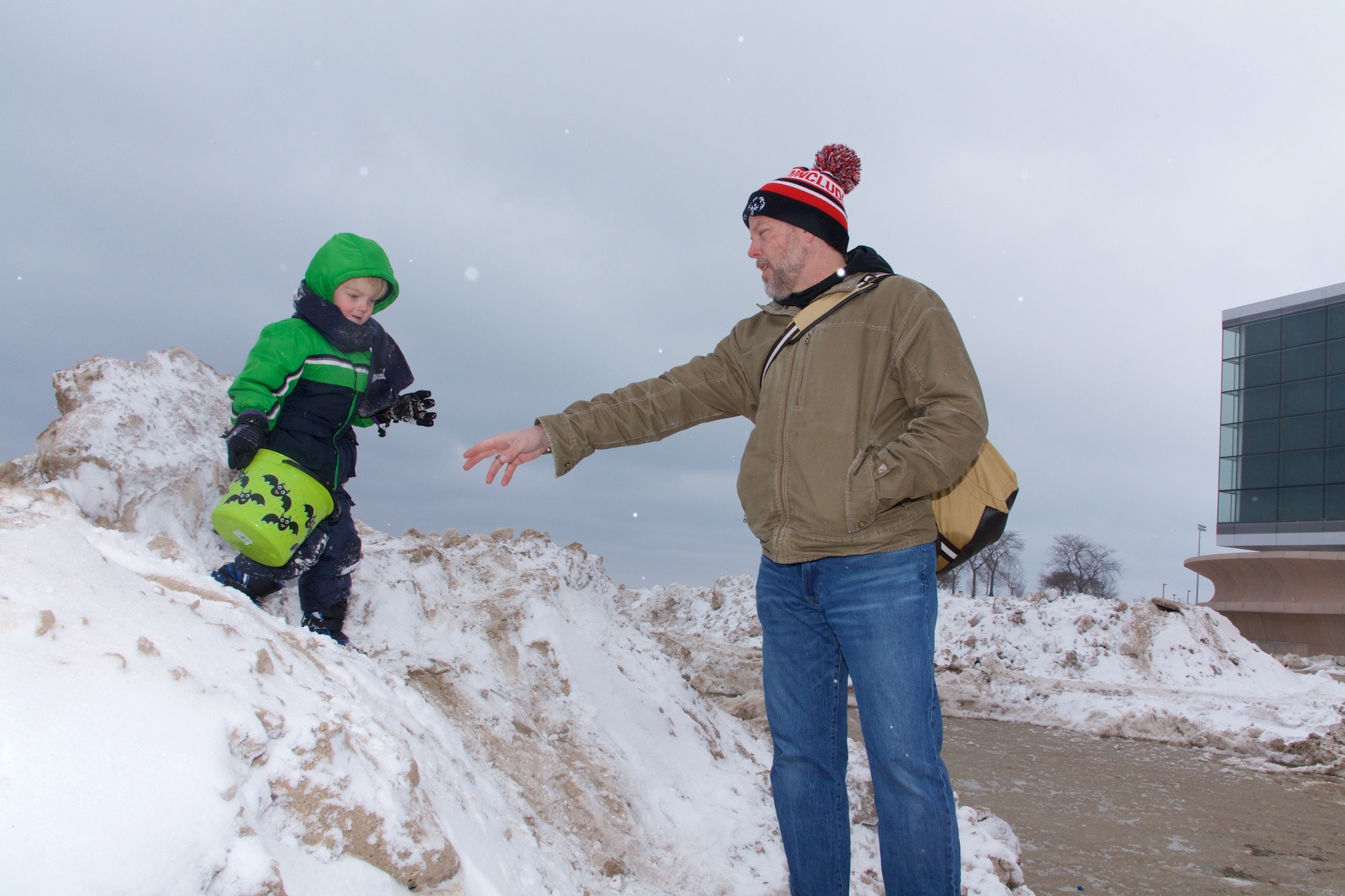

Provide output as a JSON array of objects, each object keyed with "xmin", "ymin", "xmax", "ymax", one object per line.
[
  {"xmin": 375, "ymin": 389, "xmax": 437, "ymax": 427},
  {"xmin": 226, "ymin": 423, "xmax": 266, "ymax": 470}
]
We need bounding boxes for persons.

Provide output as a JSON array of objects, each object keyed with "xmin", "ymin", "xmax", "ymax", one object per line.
[
  {"xmin": 463, "ymin": 177, "xmax": 987, "ymax": 896},
  {"xmin": 205, "ymin": 232, "xmax": 440, "ymax": 657}
]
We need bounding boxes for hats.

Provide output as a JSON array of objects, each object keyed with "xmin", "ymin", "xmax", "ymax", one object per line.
[{"xmin": 742, "ymin": 143, "xmax": 862, "ymax": 256}]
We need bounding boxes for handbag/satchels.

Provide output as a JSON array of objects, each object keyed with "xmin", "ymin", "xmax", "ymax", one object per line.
[{"xmin": 760, "ymin": 273, "xmax": 1020, "ymax": 577}]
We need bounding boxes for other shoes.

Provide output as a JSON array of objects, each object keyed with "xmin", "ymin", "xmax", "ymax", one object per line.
[{"xmin": 213, "ymin": 561, "xmax": 369, "ymax": 657}]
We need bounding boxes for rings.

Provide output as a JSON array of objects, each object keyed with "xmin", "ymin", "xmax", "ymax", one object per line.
[{"xmin": 494, "ymin": 454, "xmax": 505, "ymax": 465}]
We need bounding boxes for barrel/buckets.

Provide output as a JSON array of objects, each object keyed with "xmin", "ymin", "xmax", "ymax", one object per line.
[{"xmin": 211, "ymin": 448, "xmax": 335, "ymax": 567}]
